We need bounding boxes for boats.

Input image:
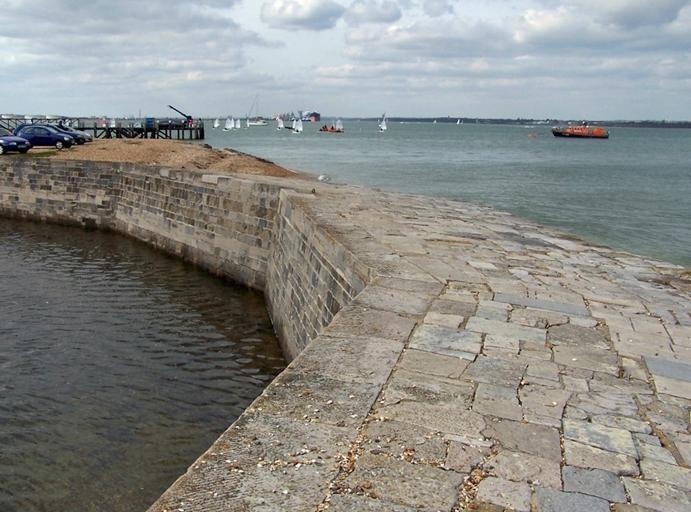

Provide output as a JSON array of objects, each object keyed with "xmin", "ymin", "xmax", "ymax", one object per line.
[
  {"xmin": 249, "ymin": 119, "xmax": 268, "ymax": 126},
  {"xmin": 551, "ymin": 123, "xmax": 609, "ymax": 139}
]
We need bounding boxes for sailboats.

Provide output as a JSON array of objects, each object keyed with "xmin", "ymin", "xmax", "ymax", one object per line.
[
  {"xmin": 212, "ymin": 117, "xmax": 221, "ymax": 127},
  {"xmin": 319, "ymin": 116, "xmax": 344, "ymax": 132},
  {"xmin": 222, "ymin": 116, "xmax": 240, "ymax": 131},
  {"xmin": 377, "ymin": 111, "xmax": 386, "ymax": 131},
  {"xmin": 276, "ymin": 117, "xmax": 303, "ymax": 134}
]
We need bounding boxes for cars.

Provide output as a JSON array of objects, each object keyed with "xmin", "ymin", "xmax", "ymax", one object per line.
[
  {"xmin": 0, "ymin": 126, "xmax": 31, "ymax": 155},
  {"xmin": 15, "ymin": 122, "xmax": 94, "ymax": 149}
]
